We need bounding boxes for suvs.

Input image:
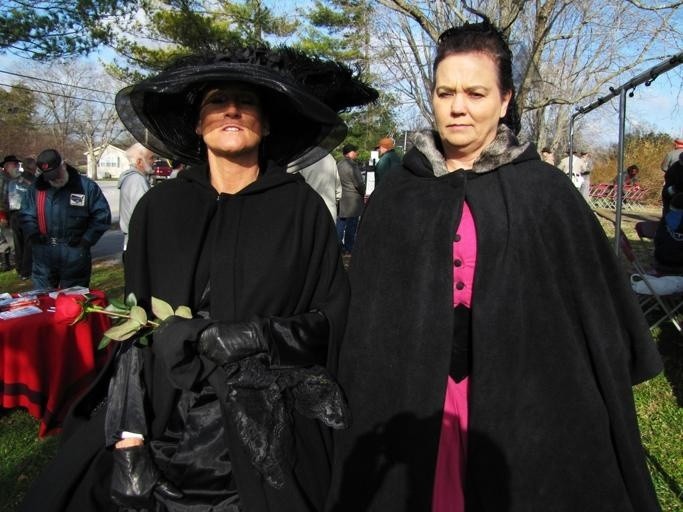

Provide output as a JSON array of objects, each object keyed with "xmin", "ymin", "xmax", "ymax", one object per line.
[{"xmin": 148, "ymin": 159, "xmax": 172, "ymax": 185}]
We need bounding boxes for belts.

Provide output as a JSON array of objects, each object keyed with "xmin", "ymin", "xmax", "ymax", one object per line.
[{"xmin": 567, "ymin": 173, "xmax": 580, "ymax": 176}]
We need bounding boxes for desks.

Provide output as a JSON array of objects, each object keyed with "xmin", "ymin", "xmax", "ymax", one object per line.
[{"xmin": 1, "ymin": 289, "xmax": 108, "ymax": 440}]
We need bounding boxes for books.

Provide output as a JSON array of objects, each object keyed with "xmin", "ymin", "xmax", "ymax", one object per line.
[{"xmin": 48, "ymin": 285, "xmax": 91, "ymax": 300}]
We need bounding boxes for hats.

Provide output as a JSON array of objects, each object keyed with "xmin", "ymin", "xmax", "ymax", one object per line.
[
  {"xmin": 343, "ymin": 144, "xmax": 359, "ymax": 155},
  {"xmin": 36, "ymin": 150, "xmax": 61, "ymax": 180},
  {"xmin": 541, "ymin": 147, "xmax": 551, "ymax": 153},
  {"xmin": 375, "ymin": 138, "xmax": 394, "ymax": 149},
  {"xmin": 563, "ymin": 148, "xmax": 587, "ymax": 155},
  {"xmin": 116, "ymin": 42, "xmax": 379, "ymax": 174},
  {"xmin": 0, "ymin": 155, "xmax": 22, "ymax": 168}
]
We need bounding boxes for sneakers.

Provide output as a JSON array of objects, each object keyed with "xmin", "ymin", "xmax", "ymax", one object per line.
[{"xmin": 22, "ymin": 275, "xmax": 30, "ymax": 280}]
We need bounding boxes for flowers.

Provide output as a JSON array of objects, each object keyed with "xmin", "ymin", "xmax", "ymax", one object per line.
[{"xmin": 52, "ymin": 292, "xmax": 193, "ymax": 351}]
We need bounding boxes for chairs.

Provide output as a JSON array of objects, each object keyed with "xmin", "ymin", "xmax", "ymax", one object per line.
[
  {"xmin": 586, "ymin": 184, "xmax": 648, "ymax": 212},
  {"xmin": 619, "ymin": 231, "xmax": 674, "ymax": 310},
  {"xmin": 635, "ymin": 221, "xmax": 681, "ymax": 317}
]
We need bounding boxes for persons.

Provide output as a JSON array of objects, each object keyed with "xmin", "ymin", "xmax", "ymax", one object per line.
[
  {"xmin": 293, "ymin": 135, "xmax": 401, "ymax": 255},
  {"xmin": 19, "ymin": 44, "xmax": 381, "ymax": 512},
  {"xmin": 323, "ymin": 18, "xmax": 667, "ymax": 510},
  {"xmin": 541, "ymin": 136, "xmax": 683, "ymax": 312},
  {"xmin": 0, "ymin": 148, "xmax": 112, "ymax": 292},
  {"xmin": 117, "ymin": 142, "xmax": 156, "ymax": 304}
]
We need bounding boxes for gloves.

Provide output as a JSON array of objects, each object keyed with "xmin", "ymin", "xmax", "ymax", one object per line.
[
  {"xmin": 74, "ymin": 238, "xmax": 91, "ymax": 257},
  {"xmin": 196, "ymin": 317, "xmax": 267, "ymax": 365},
  {"xmin": 29, "ymin": 233, "xmax": 44, "ymax": 245},
  {"xmin": 110, "ymin": 445, "xmax": 184, "ymax": 511}
]
